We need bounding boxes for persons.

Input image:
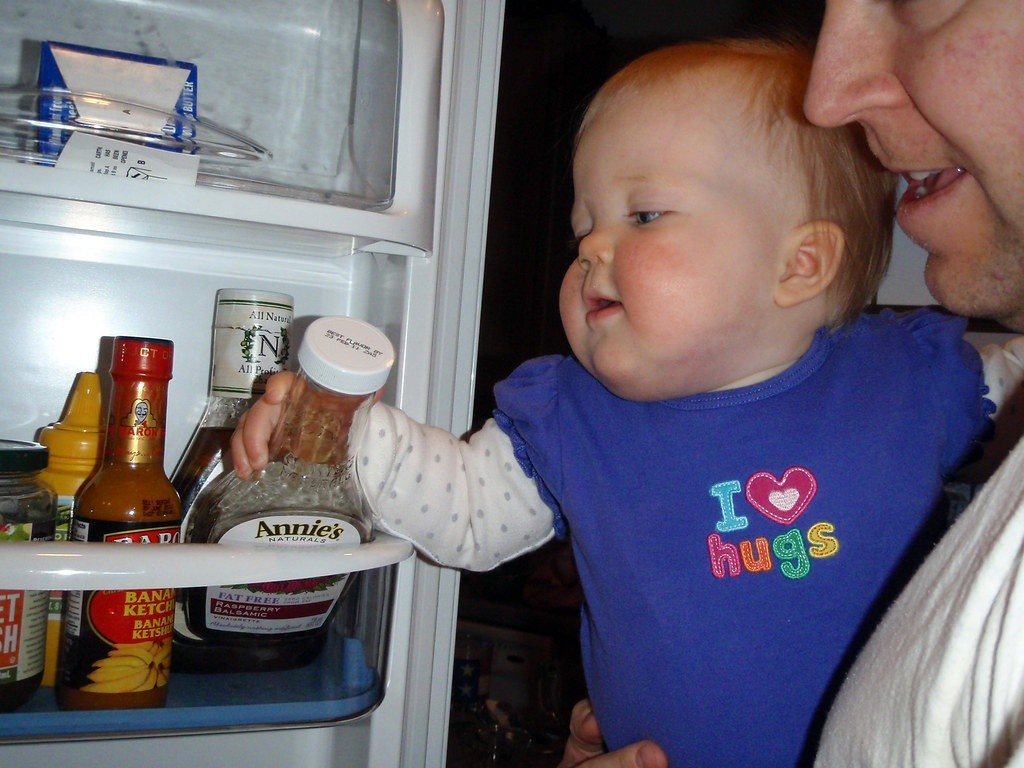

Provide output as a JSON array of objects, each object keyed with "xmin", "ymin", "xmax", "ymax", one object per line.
[
  {"xmin": 556, "ymin": 0, "xmax": 1024, "ymax": 767},
  {"xmin": 231, "ymin": 39, "xmax": 1024, "ymax": 768}
]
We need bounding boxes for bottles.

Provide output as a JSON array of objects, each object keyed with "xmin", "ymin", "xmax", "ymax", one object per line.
[
  {"xmin": 170, "ymin": 288, "xmax": 292, "ymax": 667},
  {"xmin": 0, "ymin": 439, "xmax": 59, "ymax": 713},
  {"xmin": 53, "ymin": 335, "xmax": 189, "ymax": 709},
  {"xmin": 183, "ymin": 315, "xmax": 397, "ymax": 669},
  {"xmin": 32, "ymin": 371, "xmax": 107, "ymax": 690}
]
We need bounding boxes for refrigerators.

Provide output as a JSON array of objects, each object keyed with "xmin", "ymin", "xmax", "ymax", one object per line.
[{"xmin": 0, "ymin": 0, "xmax": 504, "ymax": 768}]
